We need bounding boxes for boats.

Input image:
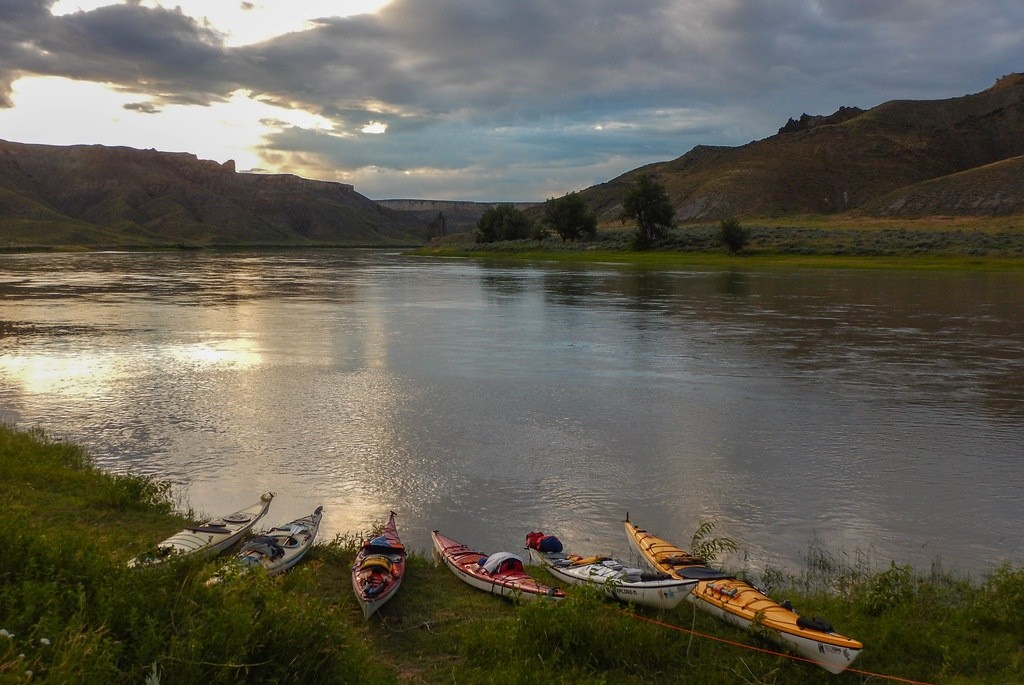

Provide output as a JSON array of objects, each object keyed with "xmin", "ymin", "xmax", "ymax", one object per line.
[
  {"xmin": 352, "ymin": 510, "xmax": 406, "ymax": 619},
  {"xmin": 526, "ymin": 531, "xmax": 699, "ymax": 611},
  {"xmin": 127, "ymin": 494, "xmax": 274, "ymax": 570},
  {"xmin": 205, "ymin": 505, "xmax": 322, "ymax": 585},
  {"xmin": 624, "ymin": 512, "xmax": 865, "ymax": 674},
  {"xmin": 431, "ymin": 531, "xmax": 565, "ymax": 602}
]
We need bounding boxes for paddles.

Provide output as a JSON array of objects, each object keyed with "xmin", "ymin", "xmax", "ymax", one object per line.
[{"xmin": 282, "ymin": 524, "xmax": 304, "ymax": 547}]
